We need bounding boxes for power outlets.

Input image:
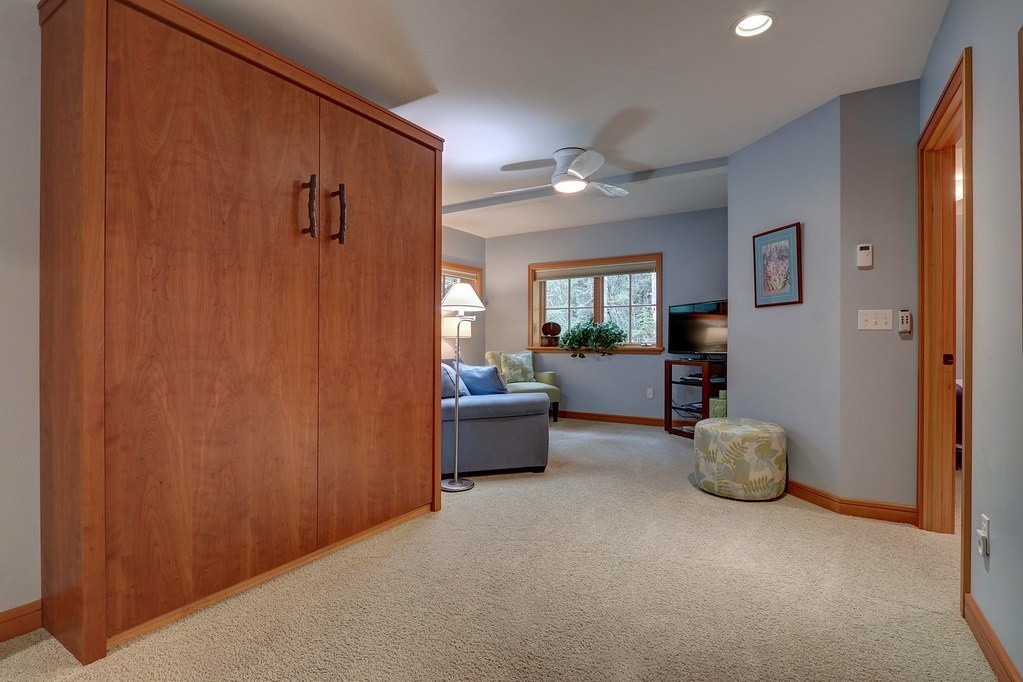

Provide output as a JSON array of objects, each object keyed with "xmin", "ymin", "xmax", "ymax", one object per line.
[
  {"xmin": 647, "ymin": 388, "xmax": 654, "ymax": 399},
  {"xmin": 980, "ymin": 514, "xmax": 991, "ymax": 555}
]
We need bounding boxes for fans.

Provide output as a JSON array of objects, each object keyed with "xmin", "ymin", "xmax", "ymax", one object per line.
[{"xmin": 492, "ymin": 148, "xmax": 629, "ymax": 197}]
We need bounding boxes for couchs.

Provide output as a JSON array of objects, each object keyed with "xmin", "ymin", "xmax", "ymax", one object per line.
[
  {"xmin": 485, "ymin": 351, "xmax": 561, "ymax": 421},
  {"xmin": 440, "ymin": 363, "xmax": 550, "ymax": 478}
]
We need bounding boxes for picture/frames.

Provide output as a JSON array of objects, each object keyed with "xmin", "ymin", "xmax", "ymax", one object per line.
[{"xmin": 752, "ymin": 221, "xmax": 804, "ymax": 308}]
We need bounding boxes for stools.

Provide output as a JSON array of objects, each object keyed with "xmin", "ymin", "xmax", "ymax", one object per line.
[{"xmin": 693, "ymin": 416, "xmax": 787, "ymax": 501}]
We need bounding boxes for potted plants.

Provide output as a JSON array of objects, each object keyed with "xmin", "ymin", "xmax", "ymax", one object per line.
[{"xmin": 557, "ymin": 314, "xmax": 629, "ymax": 358}]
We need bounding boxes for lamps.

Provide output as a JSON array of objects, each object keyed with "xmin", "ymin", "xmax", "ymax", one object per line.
[
  {"xmin": 441, "ymin": 283, "xmax": 487, "ymax": 490},
  {"xmin": 552, "ymin": 173, "xmax": 590, "ymax": 193},
  {"xmin": 442, "ymin": 316, "xmax": 472, "ymax": 359}
]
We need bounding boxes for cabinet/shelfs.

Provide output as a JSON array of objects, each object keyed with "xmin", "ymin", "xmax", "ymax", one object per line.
[
  {"xmin": 38, "ymin": 0, "xmax": 445, "ymax": 662},
  {"xmin": 665, "ymin": 359, "xmax": 727, "ymax": 438}
]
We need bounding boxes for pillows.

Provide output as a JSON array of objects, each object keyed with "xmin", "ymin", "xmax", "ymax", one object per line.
[
  {"xmin": 498, "ymin": 350, "xmax": 537, "ymax": 382},
  {"xmin": 452, "ymin": 360, "xmax": 508, "ymax": 395}
]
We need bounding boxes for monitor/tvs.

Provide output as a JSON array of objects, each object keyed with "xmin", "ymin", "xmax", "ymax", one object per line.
[{"xmin": 668, "ymin": 299, "xmax": 728, "ymax": 361}]
